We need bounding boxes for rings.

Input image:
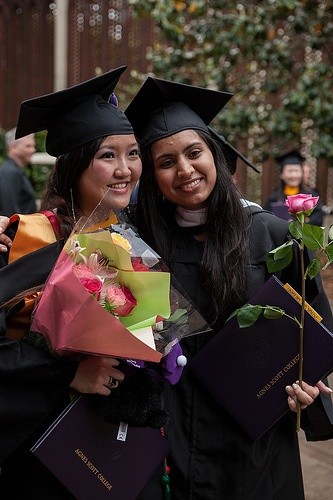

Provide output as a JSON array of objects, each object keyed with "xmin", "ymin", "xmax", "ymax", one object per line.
[{"xmin": 105, "ymin": 377, "xmax": 116, "ymax": 388}]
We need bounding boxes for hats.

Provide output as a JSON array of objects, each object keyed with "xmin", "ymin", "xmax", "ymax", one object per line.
[
  {"xmin": 274, "ymin": 148, "xmax": 306, "ymax": 165},
  {"xmin": 15, "ymin": 65, "xmax": 135, "ymax": 158},
  {"xmin": 124, "ymin": 76, "xmax": 234, "ymax": 147}
]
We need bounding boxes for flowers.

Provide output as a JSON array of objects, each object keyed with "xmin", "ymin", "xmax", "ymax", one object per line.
[
  {"xmin": 223, "ymin": 193, "xmax": 333, "ymax": 431},
  {"xmin": 29, "ymin": 187, "xmax": 212, "ymax": 409}
]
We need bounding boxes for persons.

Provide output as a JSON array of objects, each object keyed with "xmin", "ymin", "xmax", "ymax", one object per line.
[
  {"xmin": 0, "ymin": 126, "xmax": 37, "ymax": 216},
  {"xmin": 0, "ymin": 66, "xmax": 169, "ymax": 483},
  {"xmin": 0, "ymin": 77, "xmax": 332, "ymax": 499},
  {"xmin": 260, "ymin": 148, "xmax": 324, "ymax": 226}
]
